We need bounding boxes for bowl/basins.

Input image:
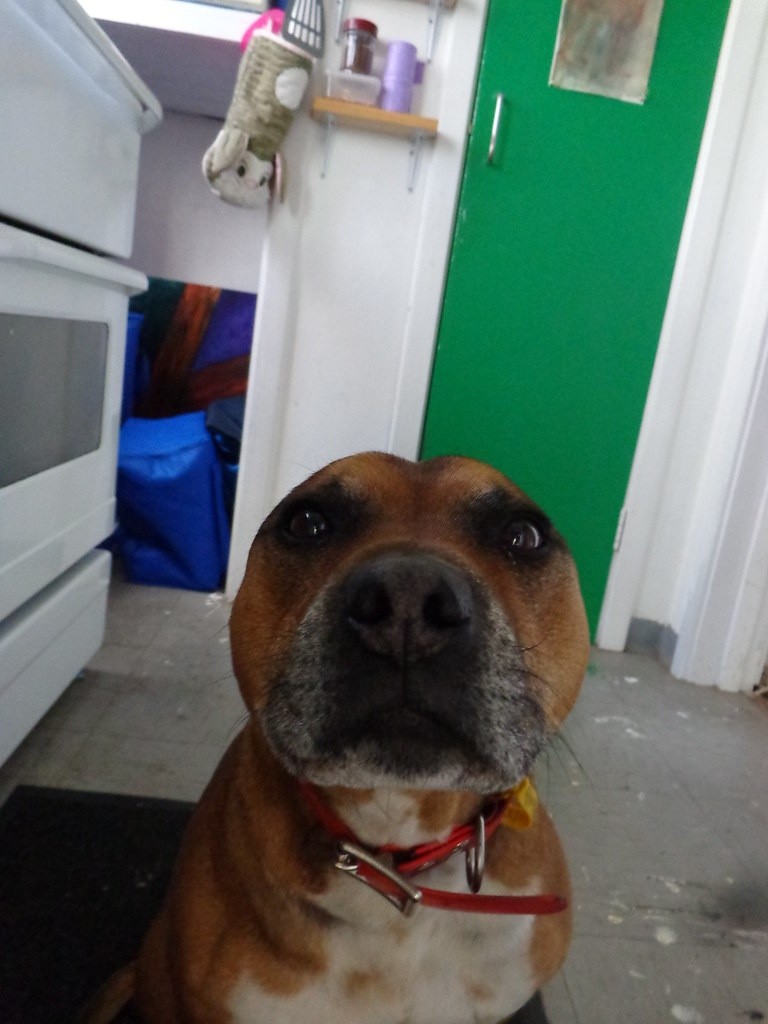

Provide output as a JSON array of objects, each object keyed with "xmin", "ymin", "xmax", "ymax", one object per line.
[{"xmin": 322, "ymin": 69, "xmax": 385, "ymax": 105}]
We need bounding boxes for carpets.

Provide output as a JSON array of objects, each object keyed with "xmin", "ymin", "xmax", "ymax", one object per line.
[{"xmin": 0, "ymin": 784, "xmax": 549, "ymax": 1024}]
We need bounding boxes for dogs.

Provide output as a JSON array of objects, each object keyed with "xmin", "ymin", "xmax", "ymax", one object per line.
[{"xmin": 1, "ymin": 449, "xmax": 590, "ymax": 1023}]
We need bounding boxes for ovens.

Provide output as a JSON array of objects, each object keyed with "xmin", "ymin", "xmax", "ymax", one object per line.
[{"xmin": 0, "ymin": 0, "xmax": 164, "ymax": 769}]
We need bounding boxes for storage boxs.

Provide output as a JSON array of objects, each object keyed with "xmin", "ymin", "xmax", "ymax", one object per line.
[{"xmin": 323, "ymin": 71, "xmax": 383, "ymax": 106}]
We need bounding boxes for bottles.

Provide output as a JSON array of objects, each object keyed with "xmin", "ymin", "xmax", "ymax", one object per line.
[
  {"xmin": 381, "ymin": 40, "xmax": 417, "ymax": 114},
  {"xmin": 340, "ymin": 19, "xmax": 378, "ymax": 74}
]
